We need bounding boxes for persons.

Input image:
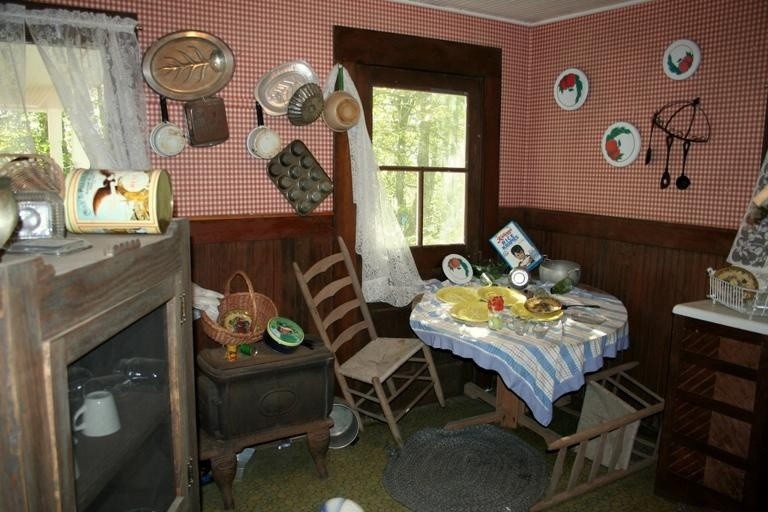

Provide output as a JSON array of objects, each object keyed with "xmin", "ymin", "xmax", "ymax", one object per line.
[{"xmin": 511, "ymin": 245, "xmax": 534, "ymax": 267}]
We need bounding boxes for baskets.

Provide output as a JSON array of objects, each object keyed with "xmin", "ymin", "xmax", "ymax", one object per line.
[
  {"xmin": 707, "ymin": 271, "xmax": 767, "ymax": 320},
  {"xmin": 199, "ymin": 271, "xmax": 278, "ymax": 347},
  {"xmin": 1, "ymin": 155, "xmax": 65, "ymax": 203}
]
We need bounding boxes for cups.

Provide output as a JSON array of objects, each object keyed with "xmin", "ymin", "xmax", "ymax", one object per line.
[
  {"xmin": 73, "ymin": 392, "xmax": 121, "ymax": 438},
  {"xmin": 66, "ymin": 356, "xmax": 172, "ymax": 394},
  {"xmin": 506, "ymin": 317, "xmax": 550, "ymax": 338},
  {"xmin": 488, "ymin": 296, "xmax": 503, "ymax": 331}
]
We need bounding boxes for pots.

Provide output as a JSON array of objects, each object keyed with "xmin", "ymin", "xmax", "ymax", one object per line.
[
  {"xmin": 321, "ymin": 66, "xmax": 363, "ymax": 133},
  {"xmin": 539, "ymin": 259, "xmax": 582, "ymax": 288},
  {"xmin": 246, "ymin": 102, "xmax": 282, "ymax": 160},
  {"xmin": 149, "ymin": 95, "xmax": 187, "ymax": 157},
  {"xmin": 524, "ymin": 295, "xmax": 599, "ymax": 317}
]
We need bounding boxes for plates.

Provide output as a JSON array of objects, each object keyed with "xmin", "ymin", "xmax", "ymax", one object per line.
[
  {"xmin": 140, "ymin": 30, "xmax": 236, "ymax": 100},
  {"xmin": 443, "ymin": 254, "xmax": 474, "ymax": 286},
  {"xmin": 184, "ymin": 96, "xmax": 229, "ymax": 148},
  {"xmin": 712, "ymin": 268, "xmax": 759, "ymax": 304},
  {"xmin": 218, "ymin": 308, "xmax": 261, "ymax": 333},
  {"xmin": 252, "ymin": 59, "xmax": 320, "ymax": 117},
  {"xmin": 436, "ymin": 287, "xmax": 564, "ymax": 325},
  {"xmin": 265, "ymin": 141, "xmax": 335, "ymax": 218}
]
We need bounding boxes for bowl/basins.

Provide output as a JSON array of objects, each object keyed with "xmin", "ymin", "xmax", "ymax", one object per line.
[
  {"xmin": 507, "ymin": 266, "xmax": 531, "ymax": 290},
  {"xmin": 286, "ymin": 83, "xmax": 324, "ymax": 126},
  {"xmin": 0, "ymin": 176, "xmax": 20, "ymax": 250},
  {"xmin": 328, "ymin": 403, "xmax": 361, "ymax": 452}
]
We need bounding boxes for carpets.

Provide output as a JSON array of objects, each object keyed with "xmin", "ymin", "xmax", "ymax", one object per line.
[{"xmin": 379, "ymin": 423, "xmax": 549, "ymax": 511}]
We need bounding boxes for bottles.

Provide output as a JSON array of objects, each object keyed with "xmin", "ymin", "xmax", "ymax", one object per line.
[
  {"xmin": 227, "ymin": 343, "xmax": 237, "ymax": 363},
  {"xmin": 472, "ymin": 259, "xmax": 499, "ymax": 286}
]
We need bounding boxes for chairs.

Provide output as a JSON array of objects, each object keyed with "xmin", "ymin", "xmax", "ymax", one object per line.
[
  {"xmin": 291, "ymin": 234, "xmax": 447, "ymax": 449},
  {"xmin": 524, "ymin": 359, "xmax": 667, "ymax": 511}
]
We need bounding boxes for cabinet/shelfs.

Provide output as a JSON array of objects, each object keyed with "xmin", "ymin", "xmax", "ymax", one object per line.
[
  {"xmin": 653, "ymin": 314, "xmax": 768, "ymax": 512},
  {"xmin": 1, "ymin": 216, "xmax": 203, "ymax": 510}
]
